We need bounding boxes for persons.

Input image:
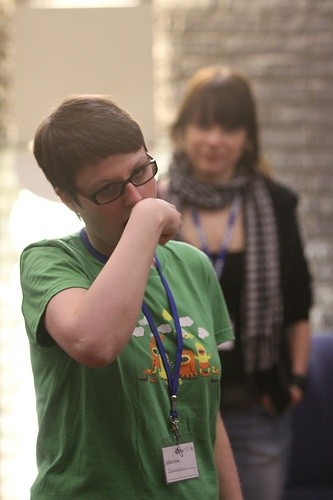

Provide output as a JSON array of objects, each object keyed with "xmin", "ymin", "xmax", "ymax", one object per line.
[
  {"xmin": 20, "ymin": 94, "xmax": 244, "ymax": 500},
  {"xmin": 153, "ymin": 64, "xmax": 312, "ymax": 500}
]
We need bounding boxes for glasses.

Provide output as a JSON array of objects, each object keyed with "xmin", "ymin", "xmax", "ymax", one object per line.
[{"xmin": 67, "ymin": 152, "xmax": 158, "ymax": 205}]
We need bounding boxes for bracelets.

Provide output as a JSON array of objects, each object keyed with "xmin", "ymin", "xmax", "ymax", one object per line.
[{"xmin": 289, "ymin": 373, "xmax": 309, "ymax": 392}]
